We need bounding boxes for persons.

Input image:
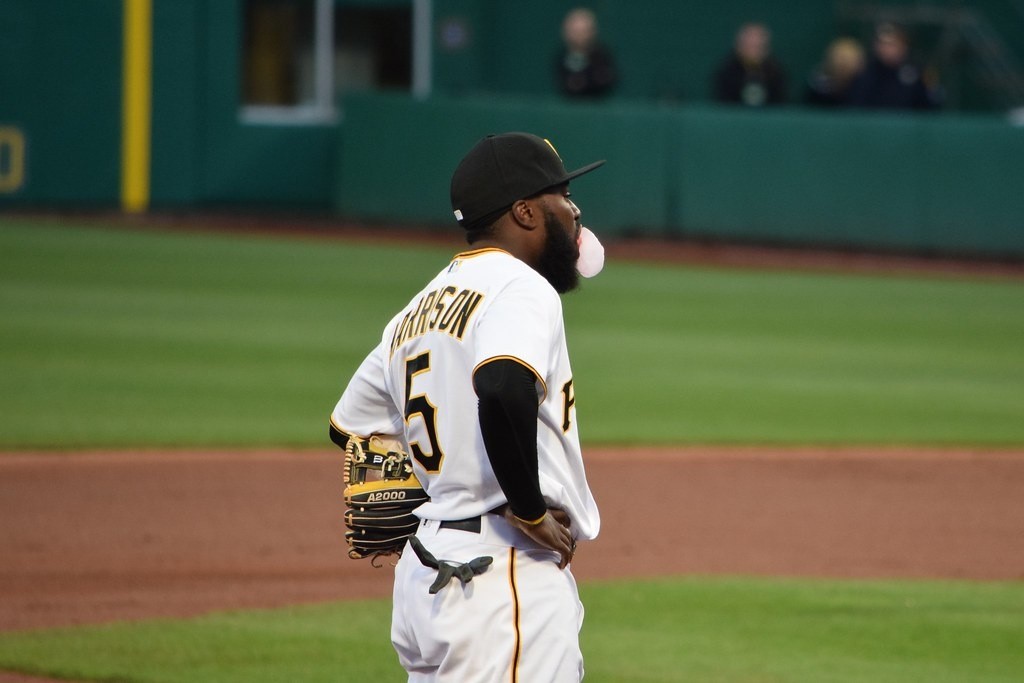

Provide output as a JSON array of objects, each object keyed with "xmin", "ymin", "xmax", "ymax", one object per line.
[
  {"xmin": 329, "ymin": 133, "xmax": 606, "ymax": 683},
  {"xmin": 553, "ymin": 11, "xmax": 617, "ymax": 100},
  {"xmin": 716, "ymin": 27, "xmax": 791, "ymax": 107},
  {"xmin": 804, "ymin": 24, "xmax": 937, "ymax": 109}
]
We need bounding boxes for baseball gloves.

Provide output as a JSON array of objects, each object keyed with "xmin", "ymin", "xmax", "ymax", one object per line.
[{"xmin": 343, "ymin": 439, "xmax": 432, "ymax": 562}]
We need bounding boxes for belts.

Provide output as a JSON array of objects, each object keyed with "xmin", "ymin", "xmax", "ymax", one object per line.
[{"xmin": 424, "ymin": 515, "xmax": 481, "ymax": 534}]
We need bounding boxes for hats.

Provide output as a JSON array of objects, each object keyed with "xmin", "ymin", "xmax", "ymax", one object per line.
[{"xmin": 451, "ymin": 132, "xmax": 606, "ymax": 225}]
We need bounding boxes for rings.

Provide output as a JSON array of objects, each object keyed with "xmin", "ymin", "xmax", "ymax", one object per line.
[{"xmin": 570, "ymin": 542, "xmax": 576, "ymax": 550}]
